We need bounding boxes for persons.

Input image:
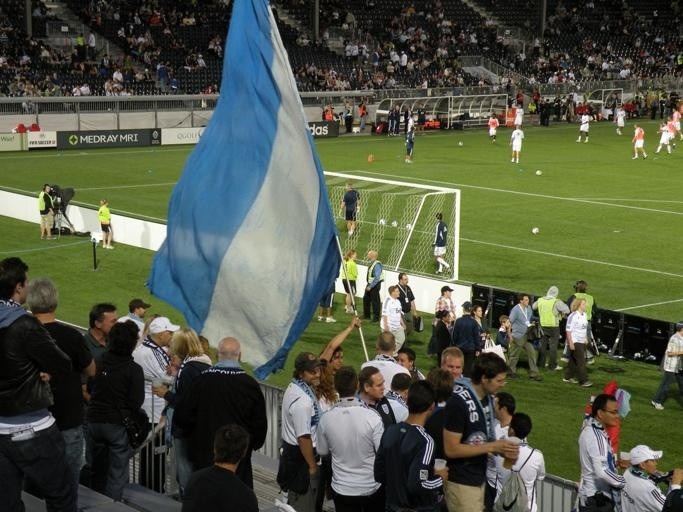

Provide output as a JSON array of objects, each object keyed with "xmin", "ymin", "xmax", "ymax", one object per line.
[
  {"xmin": 0, "ymin": 250, "xmax": 683, "ymax": 511},
  {"xmin": 430, "ymin": 214, "xmax": 451, "ymax": 275},
  {"xmin": 631, "ymin": 124, "xmax": 648, "ymax": 160},
  {"xmin": 338, "ymin": 183, "xmax": 360, "ymax": 238},
  {"xmin": 37, "ymin": 182, "xmax": 57, "ymax": 240},
  {"xmin": 509, "ymin": 125, "xmax": 525, "ymax": 164},
  {"xmin": 652, "ymin": 120, "xmax": 671, "ymax": 155},
  {"xmin": 404, "ymin": 127, "xmax": 415, "ymax": 164},
  {"xmin": 98, "ymin": 199, "xmax": 115, "ymax": 250},
  {"xmin": 2, "ymin": 0, "xmax": 683, "ymax": 143}
]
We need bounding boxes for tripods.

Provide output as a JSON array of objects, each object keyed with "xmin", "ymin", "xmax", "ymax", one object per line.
[{"xmin": 563, "ymin": 329, "xmax": 600, "ymax": 359}]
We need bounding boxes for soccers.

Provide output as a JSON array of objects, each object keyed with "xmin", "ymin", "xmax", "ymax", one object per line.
[
  {"xmin": 368, "ymin": 155, "xmax": 374, "ymax": 162},
  {"xmin": 458, "ymin": 141, "xmax": 463, "ymax": 146},
  {"xmin": 532, "ymin": 228, "xmax": 539, "ymax": 234},
  {"xmin": 406, "ymin": 224, "xmax": 412, "ymax": 230},
  {"xmin": 536, "ymin": 170, "xmax": 543, "ymax": 176},
  {"xmin": 380, "ymin": 219, "xmax": 386, "ymax": 225},
  {"xmin": 392, "ymin": 220, "xmax": 398, "ymax": 227}
]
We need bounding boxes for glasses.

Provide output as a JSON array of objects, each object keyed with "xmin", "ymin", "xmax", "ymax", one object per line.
[{"xmin": 601, "ymin": 409, "xmax": 619, "ymax": 415}]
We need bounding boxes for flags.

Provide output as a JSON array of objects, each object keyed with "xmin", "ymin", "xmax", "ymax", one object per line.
[{"xmin": 145, "ymin": 0, "xmax": 341, "ymax": 381}]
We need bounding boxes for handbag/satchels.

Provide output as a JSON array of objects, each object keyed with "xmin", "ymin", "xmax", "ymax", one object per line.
[
  {"xmin": 527, "ymin": 321, "xmax": 544, "ymax": 340},
  {"xmin": 414, "ymin": 315, "xmax": 424, "ymax": 333},
  {"xmin": 482, "ymin": 337, "xmax": 507, "ymax": 364},
  {"xmin": 122, "ymin": 408, "xmax": 149, "ymax": 449}
]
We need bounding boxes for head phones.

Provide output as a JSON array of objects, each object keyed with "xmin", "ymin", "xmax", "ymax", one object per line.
[{"xmin": 573, "ymin": 280, "xmax": 585, "ymax": 291}]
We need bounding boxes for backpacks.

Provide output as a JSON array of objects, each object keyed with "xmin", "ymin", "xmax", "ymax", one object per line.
[{"xmin": 493, "ymin": 449, "xmax": 534, "ymax": 512}]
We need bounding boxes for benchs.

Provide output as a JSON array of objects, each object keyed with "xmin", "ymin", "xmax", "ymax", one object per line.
[
  {"xmin": 21, "ymin": 491, "xmax": 46, "ymax": 510},
  {"xmin": 77, "ymin": 482, "xmax": 114, "ymax": 512},
  {"xmin": 83, "ymin": 500, "xmax": 141, "ymax": 512},
  {"xmin": 120, "ymin": 482, "xmax": 183, "ymax": 511}
]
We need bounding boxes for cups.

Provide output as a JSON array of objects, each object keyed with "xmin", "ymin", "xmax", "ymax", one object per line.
[
  {"xmin": 503, "ymin": 437, "xmax": 521, "ymax": 468},
  {"xmin": 151, "ymin": 379, "xmax": 161, "ymax": 394},
  {"xmin": 434, "ymin": 459, "xmax": 446, "ymax": 470},
  {"xmin": 620, "ymin": 452, "xmax": 629, "ymax": 468}
]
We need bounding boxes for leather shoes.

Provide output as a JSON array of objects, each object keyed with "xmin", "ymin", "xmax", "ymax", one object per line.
[
  {"xmin": 359, "ymin": 315, "xmax": 371, "ymax": 320},
  {"xmin": 372, "ymin": 319, "xmax": 379, "ymax": 322}
]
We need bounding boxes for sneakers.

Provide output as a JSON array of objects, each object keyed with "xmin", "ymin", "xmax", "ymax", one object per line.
[
  {"xmin": 651, "ymin": 401, "xmax": 665, "ymax": 412},
  {"xmin": 103, "ymin": 245, "xmax": 107, "ymax": 249},
  {"xmin": 40, "ymin": 235, "xmax": 44, "ymax": 240},
  {"xmin": 47, "ymin": 236, "xmax": 57, "ymax": 240},
  {"xmin": 326, "ymin": 317, "xmax": 337, "ymax": 323},
  {"xmin": 317, "ymin": 316, "xmax": 325, "ymax": 322},
  {"xmin": 107, "ymin": 246, "xmax": 114, "ymax": 250},
  {"xmin": 507, "ymin": 354, "xmax": 596, "ymax": 388}
]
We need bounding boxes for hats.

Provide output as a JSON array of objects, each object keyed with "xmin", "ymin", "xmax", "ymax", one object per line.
[
  {"xmin": 461, "ymin": 302, "xmax": 471, "ymax": 308},
  {"xmin": 629, "ymin": 444, "xmax": 664, "ymax": 466},
  {"xmin": 149, "ymin": 317, "xmax": 181, "ymax": 334},
  {"xmin": 295, "ymin": 352, "xmax": 325, "ymax": 372},
  {"xmin": 441, "ymin": 286, "xmax": 454, "ymax": 292},
  {"xmin": 129, "ymin": 299, "xmax": 151, "ymax": 309}
]
